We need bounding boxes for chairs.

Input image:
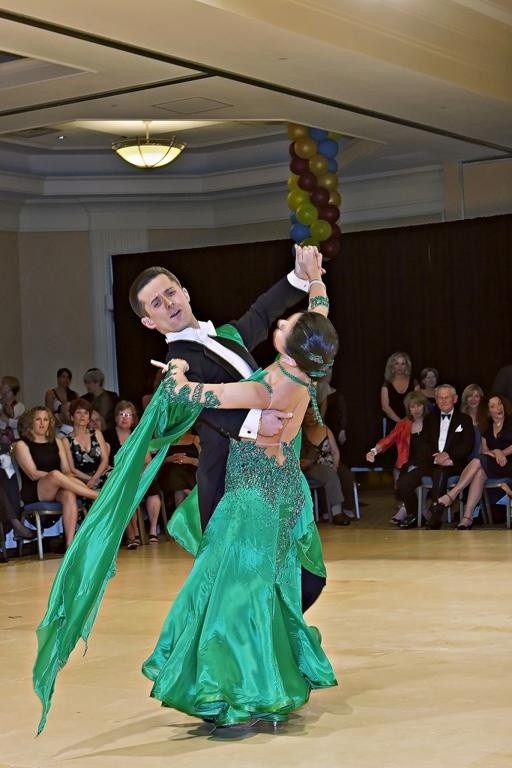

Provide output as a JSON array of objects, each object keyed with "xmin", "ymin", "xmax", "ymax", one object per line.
[
  {"xmin": 307, "ymin": 417, "xmax": 512, "ymax": 528},
  {"xmin": 11, "ymin": 451, "xmax": 167, "ymax": 560}
]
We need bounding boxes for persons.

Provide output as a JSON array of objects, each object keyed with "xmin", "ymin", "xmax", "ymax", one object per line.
[
  {"xmin": 128, "ymin": 243, "xmax": 327, "ymax": 616},
  {"xmin": 301, "ymin": 349, "xmax": 512, "ymax": 528},
  {"xmin": 161, "ymin": 241, "xmax": 340, "ymax": 735},
  {"xmin": 0, "ymin": 366, "xmax": 201, "ymax": 561}
]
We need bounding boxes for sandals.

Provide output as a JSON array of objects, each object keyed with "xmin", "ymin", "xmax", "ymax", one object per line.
[
  {"xmin": 389, "ymin": 507, "xmax": 407, "ymax": 525},
  {"xmin": 134, "ymin": 535, "xmax": 141, "ymax": 545},
  {"xmin": 430, "ymin": 495, "xmax": 454, "ymax": 512},
  {"xmin": 455, "ymin": 517, "xmax": 473, "ymax": 530},
  {"xmin": 128, "ymin": 542, "xmax": 137, "ymax": 549},
  {"xmin": 148, "ymin": 535, "xmax": 158, "ymax": 544}
]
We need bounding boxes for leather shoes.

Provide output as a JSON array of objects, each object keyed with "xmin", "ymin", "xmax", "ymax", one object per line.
[
  {"xmin": 426, "ymin": 515, "xmax": 441, "ymax": 530},
  {"xmin": 398, "ymin": 514, "xmax": 425, "ymax": 530},
  {"xmin": 15, "ymin": 527, "xmax": 37, "ymax": 539}
]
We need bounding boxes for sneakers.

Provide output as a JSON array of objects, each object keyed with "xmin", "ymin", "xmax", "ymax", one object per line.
[{"xmin": 332, "ymin": 513, "xmax": 350, "ymax": 526}]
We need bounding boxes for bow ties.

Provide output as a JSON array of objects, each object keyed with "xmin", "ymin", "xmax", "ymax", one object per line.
[{"xmin": 441, "ymin": 414, "xmax": 451, "ymax": 419}]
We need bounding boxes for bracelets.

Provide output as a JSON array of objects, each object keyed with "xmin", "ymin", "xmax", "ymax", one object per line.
[{"xmin": 308, "ymin": 279, "xmax": 327, "ymax": 289}]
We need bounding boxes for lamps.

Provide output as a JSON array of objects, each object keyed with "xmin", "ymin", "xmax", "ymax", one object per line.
[{"xmin": 111, "ymin": 119, "xmax": 186, "ymax": 169}]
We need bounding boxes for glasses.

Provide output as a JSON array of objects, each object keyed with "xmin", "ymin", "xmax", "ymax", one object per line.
[{"xmin": 120, "ymin": 413, "xmax": 133, "ymax": 417}]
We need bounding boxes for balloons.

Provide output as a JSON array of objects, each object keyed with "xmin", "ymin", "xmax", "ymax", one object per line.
[{"xmin": 285, "ymin": 120, "xmax": 341, "ymax": 257}]
